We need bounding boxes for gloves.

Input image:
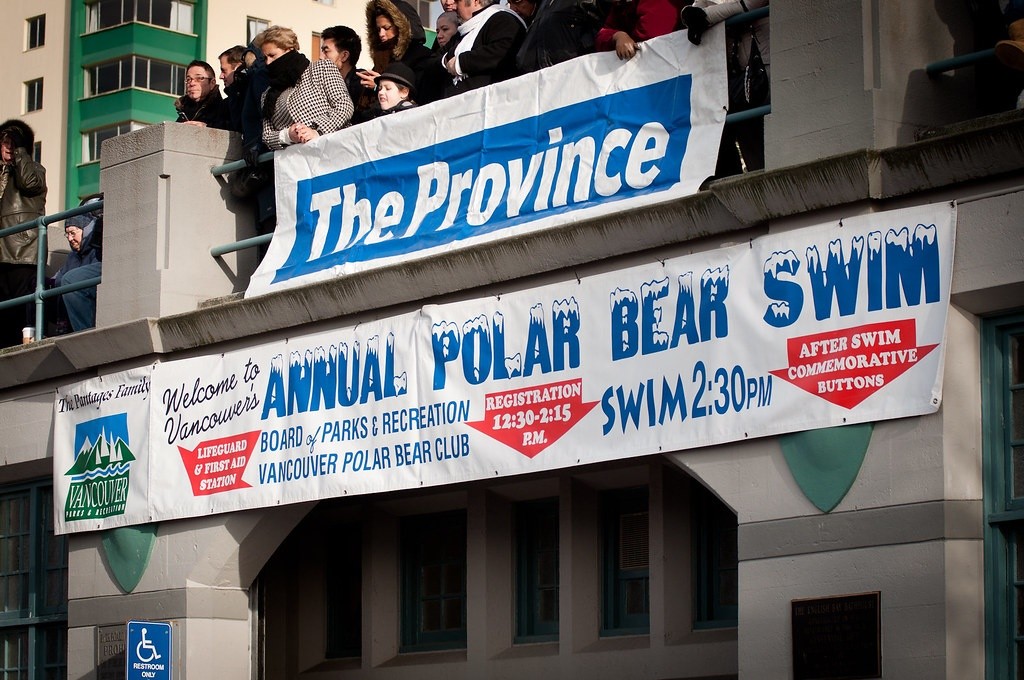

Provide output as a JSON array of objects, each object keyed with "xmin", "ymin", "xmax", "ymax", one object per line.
[
  {"xmin": 33, "ymin": 276, "xmax": 52, "ymax": 291},
  {"xmin": 5, "ymin": 126, "xmax": 26, "ymax": 148}
]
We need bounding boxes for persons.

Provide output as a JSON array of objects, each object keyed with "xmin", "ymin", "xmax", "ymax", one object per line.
[
  {"xmin": 965, "ymin": 0, "xmax": 1024, "ymax": 118},
  {"xmin": 60, "ymin": 192, "xmax": 104, "ymax": 334},
  {"xmin": 217, "ymin": 1, "xmax": 784, "ymax": 269},
  {"xmin": 175, "ymin": 60, "xmax": 225, "ymax": 129},
  {"xmin": 0, "ymin": 119, "xmax": 48, "ymax": 351},
  {"xmin": 42, "ymin": 209, "xmax": 99, "ymax": 338}
]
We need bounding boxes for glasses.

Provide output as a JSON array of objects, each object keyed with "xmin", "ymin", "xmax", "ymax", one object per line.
[{"xmin": 184, "ymin": 76, "xmax": 212, "ymax": 83}]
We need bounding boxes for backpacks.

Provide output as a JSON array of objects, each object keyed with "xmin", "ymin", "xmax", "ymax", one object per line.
[{"xmin": 727, "ymin": 62, "xmax": 746, "ymax": 113}]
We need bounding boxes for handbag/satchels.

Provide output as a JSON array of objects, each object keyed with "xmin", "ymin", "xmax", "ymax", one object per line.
[{"xmin": 744, "ymin": 38, "xmax": 769, "ymax": 108}]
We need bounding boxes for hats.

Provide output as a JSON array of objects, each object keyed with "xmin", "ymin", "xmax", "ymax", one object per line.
[
  {"xmin": 373, "ymin": 63, "xmax": 417, "ymax": 93},
  {"xmin": 65, "ymin": 215, "xmax": 93, "ymax": 229}
]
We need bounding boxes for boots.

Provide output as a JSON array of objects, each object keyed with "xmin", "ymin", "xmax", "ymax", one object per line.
[{"xmin": 995, "ymin": 18, "xmax": 1024, "ymax": 71}]
